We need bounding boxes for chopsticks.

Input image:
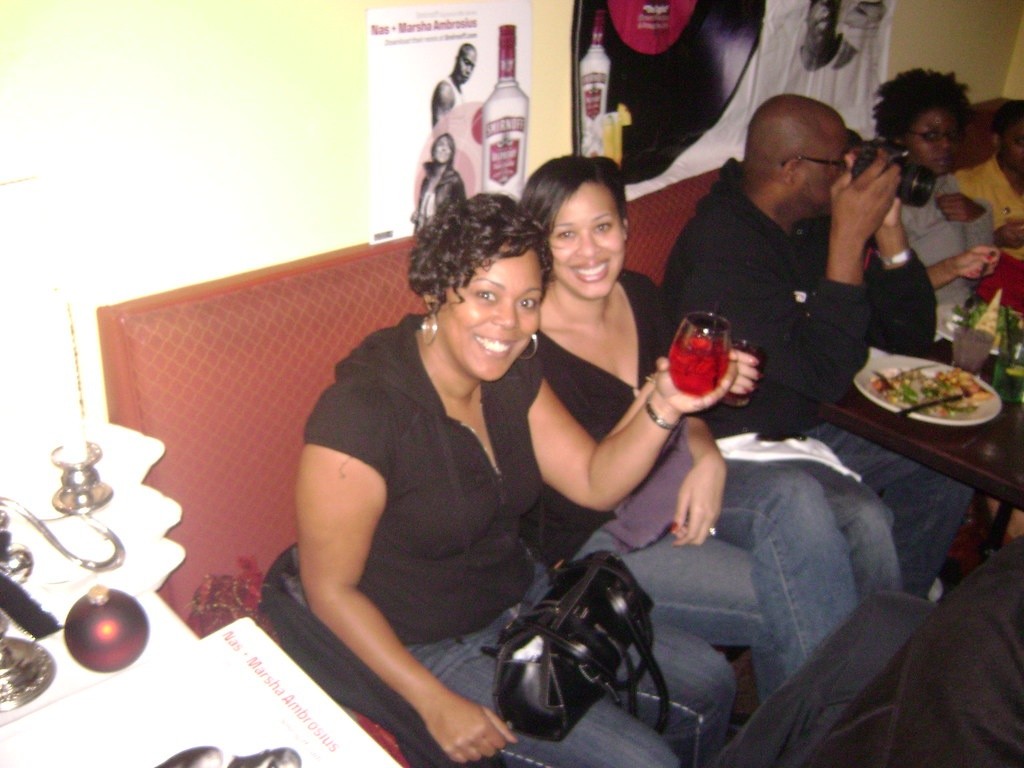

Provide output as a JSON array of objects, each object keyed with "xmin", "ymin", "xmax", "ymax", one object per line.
[{"xmin": 891, "ymin": 395, "xmax": 963, "ymax": 418}]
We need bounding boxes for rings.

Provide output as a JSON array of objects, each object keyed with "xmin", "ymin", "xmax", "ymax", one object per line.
[{"xmin": 710, "ymin": 527, "xmax": 717, "ymax": 537}]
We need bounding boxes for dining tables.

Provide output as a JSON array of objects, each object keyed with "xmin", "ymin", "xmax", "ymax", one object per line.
[{"xmin": 820, "ymin": 338, "xmax": 1024, "ymax": 578}]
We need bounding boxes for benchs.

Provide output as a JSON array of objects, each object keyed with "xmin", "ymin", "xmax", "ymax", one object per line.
[{"xmin": 97, "ymin": 95, "xmax": 1009, "ymax": 767}]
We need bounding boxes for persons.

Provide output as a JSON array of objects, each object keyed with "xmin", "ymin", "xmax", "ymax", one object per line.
[
  {"xmin": 433, "ymin": 43, "xmax": 477, "ymax": 133},
  {"xmin": 708, "ymin": 537, "xmax": 1024, "ymax": 768},
  {"xmin": 414, "ymin": 133, "xmax": 467, "ymax": 234},
  {"xmin": 521, "ymin": 155, "xmax": 860, "ymax": 705},
  {"xmin": 659, "ymin": 94, "xmax": 975, "ymax": 598},
  {"xmin": 785, "ymin": 0, "xmax": 880, "ymax": 111},
  {"xmin": 296, "ymin": 192, "xmax": 739, "ymax": 768},
  {"xmin": 873, "ymin": 67, "xmax": 1024, "ymax": 331}
]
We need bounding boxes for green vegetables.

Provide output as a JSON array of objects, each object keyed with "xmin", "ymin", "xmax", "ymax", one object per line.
[
  {"xmin": 948, "ymin": 301, "xmax": 1024, "ymax": 346},
  {"xmin": 892, "ymin": 377, "xmax": 979, "ymax": 416}
]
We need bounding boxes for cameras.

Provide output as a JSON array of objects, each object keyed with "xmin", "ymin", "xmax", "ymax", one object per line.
[{"xmin": 850, "ymin": 141, "xmax": 935, "ymax": 208}]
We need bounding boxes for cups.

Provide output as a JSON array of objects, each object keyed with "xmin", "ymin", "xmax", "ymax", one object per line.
[
  {"xmin": 669, "ymin": 314, "xmax": 733, "ymax": 397},
  {"xmin": 955, "ymin": 328, "xmax": 994, "ymax": 373},
  {"xmin": 993, "ymin": 335, "xmax": 1024, "ymax": 400}
]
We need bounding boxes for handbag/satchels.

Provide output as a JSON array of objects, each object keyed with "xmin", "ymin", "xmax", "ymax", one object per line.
[{"xmin": 480, "ymin": 548, "xmax": 670, "ymax": 742}]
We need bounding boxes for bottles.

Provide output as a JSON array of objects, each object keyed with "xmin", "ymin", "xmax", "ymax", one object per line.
[
  {"xmin": 579, "ymin": 9, "xmax": 609, "ymax": 143},
  {"xmin": 476, "ymin": 23, "xmax": 529, "ymax": 202}
]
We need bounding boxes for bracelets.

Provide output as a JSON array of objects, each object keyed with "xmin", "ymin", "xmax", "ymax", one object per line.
[
  {"xmin": 878, "ymin": 248, "xmax": 912, "ymax": 266},
  {"xmin": 647, "ymin": 394, "xmax": 679, "ymax": 431},
  {"xmin": 647, "ymin": 372, "xmax": 659, "ymax": 388}
]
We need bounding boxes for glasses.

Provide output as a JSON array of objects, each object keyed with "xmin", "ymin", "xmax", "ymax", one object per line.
[
  {"xmin": 781, "ymin": 154, "xmax": 845, "ymax": 170},
  {"xmin": 910, "ymin": 129, "xmax": 960, "ymax": 141}
]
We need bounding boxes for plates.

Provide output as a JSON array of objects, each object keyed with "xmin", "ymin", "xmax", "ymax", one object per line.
[
  {"xmin": 855, "ymin": 352, "xmax": 1000, "ymax": 426},
  {"xmin": 936, "ymin": 304, "xmax": 1001, "ymax": 355}
]
